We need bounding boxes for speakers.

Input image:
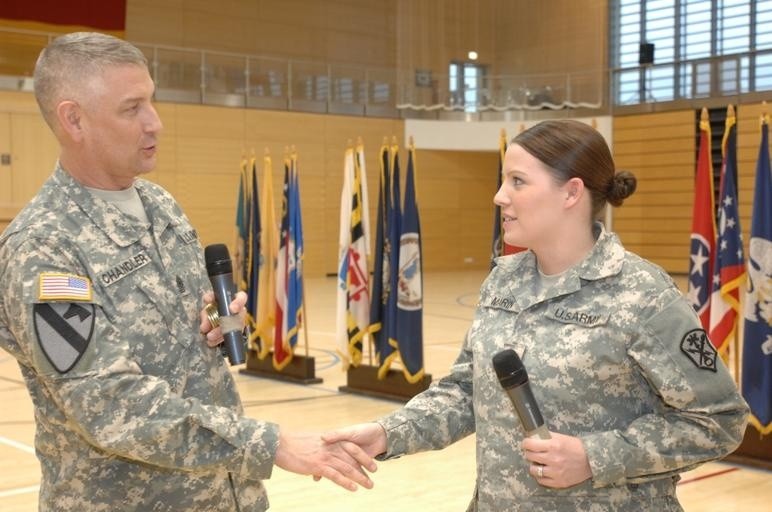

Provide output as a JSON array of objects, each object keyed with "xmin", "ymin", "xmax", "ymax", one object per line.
[{"xmin": 640, "ymin": 41, "xmax": 653, "ymax": 67}]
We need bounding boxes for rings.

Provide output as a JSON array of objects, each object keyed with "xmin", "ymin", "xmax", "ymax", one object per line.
[{"xmin": 537, "ymin": 465, "xmax": 544, "ymax": 478}]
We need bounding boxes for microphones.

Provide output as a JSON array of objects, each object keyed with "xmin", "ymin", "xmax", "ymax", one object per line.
[
  {"xmin": 493, "ymin": 349, "xmax": 552, "ymax": 444},
  {"xmin": 204, "ymin": 240, "xmax": 246, "ymax": 367}
]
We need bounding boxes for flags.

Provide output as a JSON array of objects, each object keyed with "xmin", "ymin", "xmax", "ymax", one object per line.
[
  {"xmin": 231, "ymin": 154, "xmax": 304, "ymax": 371},
  {"xmin": 735, "ymin": 100, "xmax": 771, "ymax": 437},
  {"xmin": 370, "ymin": 143, "xmax": 426, "ymax": 385},
  {"xmin": 686, "ymin": 106, "xmax": 719, "ymax": 339},
  {"xmin": 346, "ymin": 144, "xmax": 372, "ymax": 367},
  {"xmin": 706, "ymin": 103, "xmax": 746, "ymax": 367},
  {"xmin": 488, "ymin": 125, "xmax": 526, "ymax": 273},
  {"xmin": 336, "ymin": 147, "xmax": 354, "ymax": 373}
]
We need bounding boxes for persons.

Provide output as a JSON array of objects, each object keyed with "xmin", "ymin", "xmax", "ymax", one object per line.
[
  {"xmin": 1, "ymin": 30, "xmax": 380, "ymax": 512},
  {"xmin": 311, "ymin": 118, "xmax": 752, "ymax": 512}
]
